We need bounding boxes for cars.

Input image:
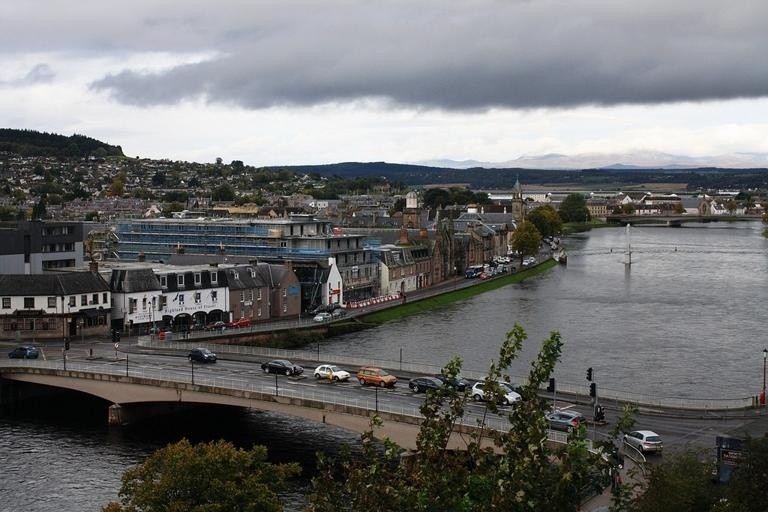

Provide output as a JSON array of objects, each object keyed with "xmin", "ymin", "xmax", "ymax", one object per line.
[
  {"xmin": 465, "ymin": 247, "xmax": 537, "ymax": 281},
  {"xmin": 188, "ymin": 347, "xmax": 217, "ymax": 365},
  {"xmin": 313, "ymin": 365, "xmax": 351, "ymax": 382},
  {"xmin": 260, "ymin": 359, "xmax": 304, "ymax": 378},
  {"xmin": 307, "ymin": 303, "xmax": 349, "ymax": 324},
  {"xmin": 543, "ymin": 235, "xmax": 560, "ymax": 250},
  {"xmin": 207, "ymin": 316, "xmax": 255, "ymax": 332}
]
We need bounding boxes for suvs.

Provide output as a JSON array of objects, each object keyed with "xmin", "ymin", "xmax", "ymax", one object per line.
[
  {"xmin": 622, "ymin": 430, "xmax": 665, "ymax": 456},
  {"xmin": 356, "ymin": 367, "xmax": 398, "ymax": 387},
  {"xmin": 407, "ymin": 372, "xmax": 523, "ymax": 408},
  {"xmin": 8, "ymin": 345, "xmax": 39, "ymax": 361},
  {"xmin": 544, "ymin": 410, "xmax": 588, "ymax": 436}
]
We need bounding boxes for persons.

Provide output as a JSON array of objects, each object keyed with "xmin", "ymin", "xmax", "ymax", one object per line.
[
  {"xmin": 613, "ymin": 471, "xmax": 623, "ymax": 493},
  {"xmin": 63, "ymin": 336, "xmax": 71, "ymax": 360}
]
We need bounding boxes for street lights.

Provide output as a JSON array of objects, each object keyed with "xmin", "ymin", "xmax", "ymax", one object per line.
[
  {"xmin": 762, "ymin": 346, "xmax": 768, "ymax": 404},
  {"xmin": 147, "ymin": 299, "xmax": 153, "ymax": 337}
]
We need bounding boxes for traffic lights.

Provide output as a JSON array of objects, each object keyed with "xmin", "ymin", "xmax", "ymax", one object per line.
[
  {"xmin": 586, "ymin": 367, "xmax": 591, "ymax": 382},
  {"xmin": 589, "ymin": 382, "xmax": 593, "ymax": 397},
  {"xmin": 598, "ymin": 404, "xmax": 606, "ymax": 422}
]
